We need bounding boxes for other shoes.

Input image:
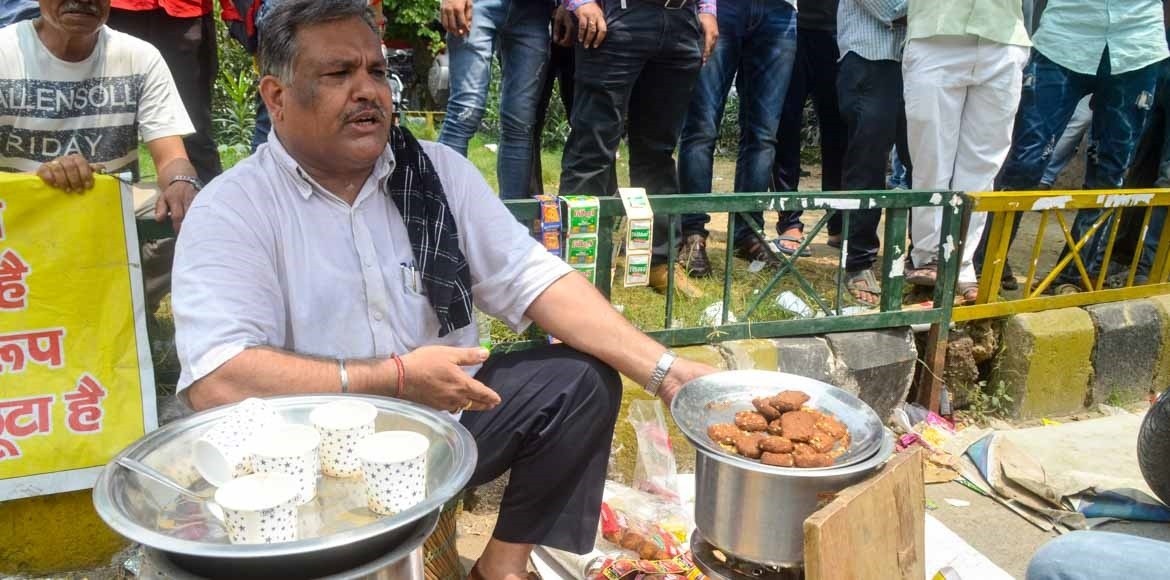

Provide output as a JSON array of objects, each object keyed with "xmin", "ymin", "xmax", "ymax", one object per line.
[{"xmin": 908, "ymin": 262, "xmax": 1153, "ymax": 307}]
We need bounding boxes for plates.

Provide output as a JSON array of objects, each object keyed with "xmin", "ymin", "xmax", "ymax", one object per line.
[{"xmin": 670, "ymin": 369, "xmax": 884, "ymax": 470}]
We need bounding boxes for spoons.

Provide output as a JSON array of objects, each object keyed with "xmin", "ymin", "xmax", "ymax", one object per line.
[{"xmin": 114, "ymin": 456, "xmax": 227, "ymax": 530}]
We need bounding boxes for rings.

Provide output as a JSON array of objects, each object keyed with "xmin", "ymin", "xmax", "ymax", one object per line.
[
  {"xmin": 588, "ymin": 20, "xmax": 595, "ymax": 25},
  {"xmin": 450, "ymin": 407, "xmax": 461, "ymax": 415},
  {"xmin": 463, "ymin": 399, "xmax": 472, "ymax": 409}
]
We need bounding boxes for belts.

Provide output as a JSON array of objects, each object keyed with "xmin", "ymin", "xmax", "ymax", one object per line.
[{"xmin": 659, "ymin": 0, "xmax": 691, "ymax": 11}]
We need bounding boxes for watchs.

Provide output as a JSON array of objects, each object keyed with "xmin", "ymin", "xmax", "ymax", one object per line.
[{"xmin": 169, "ymin": 176, "xmax": 204, "ymax": 191}]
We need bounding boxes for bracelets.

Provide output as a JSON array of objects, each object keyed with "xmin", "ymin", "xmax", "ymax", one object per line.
[
  {"xmin": 338, "ymin": 358, "xmax": 348, "ymax": 393},
  {"xmin": 391, "ymin": 352, "xmax": 403, "ymax": 398},
  {"xmin": 644, "ymin": 352, "xmax": 678, "ymax": 398}
]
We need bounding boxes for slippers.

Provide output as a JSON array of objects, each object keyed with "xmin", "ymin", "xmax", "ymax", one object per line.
[
  {"xmin": 829, "ymin": 237, "xmax": 843, "ymax": 249},
  {"xmin": 776, "ymin": 232, "xmax": 811, "ymax": 257},
  {"xmin": 845, "ymin": 268, "xmax": 883, "ymax": 308}
]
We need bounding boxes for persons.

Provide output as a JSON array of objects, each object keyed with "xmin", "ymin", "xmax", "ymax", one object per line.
[
  {"xmin": 974, "ymin": 0, "xmax": 1170, "ymax": 295},
  {"xmin": 436, "ymin": 0, "xmax": 912, "ymax": 306},
  {"xmin": 901, "ymin": 0, "xmax": 1032, "ymax": 301},
  {"xmin": 220, "ymin": 0, "xmax": 270, "ymax": 153},
  {"xmin": 171, "ymin": 0, "xmax": 719, "ymax": 579},
  {"xmin": 106, "ymin": 0, "xmax": 222, "ymax": 184},
  {"xmin": 1, "ymin": 0, "xmax": 200, "ymax": 334}
]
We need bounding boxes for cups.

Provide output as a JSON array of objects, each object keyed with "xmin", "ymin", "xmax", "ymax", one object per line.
[{"xmin": 191, "ymin": 397, "xmax": 429, "ymax": 544}]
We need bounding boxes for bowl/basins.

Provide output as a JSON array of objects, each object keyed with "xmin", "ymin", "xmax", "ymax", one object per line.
[{"xmin": 92, "ymin": 393, "xmax": 478, "ymax": 579}]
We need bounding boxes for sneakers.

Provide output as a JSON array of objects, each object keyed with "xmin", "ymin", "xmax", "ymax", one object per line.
[
  {"xmin": 651, "ymin": 263, "xmax": 702, "ymax": 301},
  {"xmin": 735, "ymin": 238, "xmax": 787, "ymax": 271},
  {"xmin": 684, "ymin": 235, "xmax": 712, "ymax": 278}
]
